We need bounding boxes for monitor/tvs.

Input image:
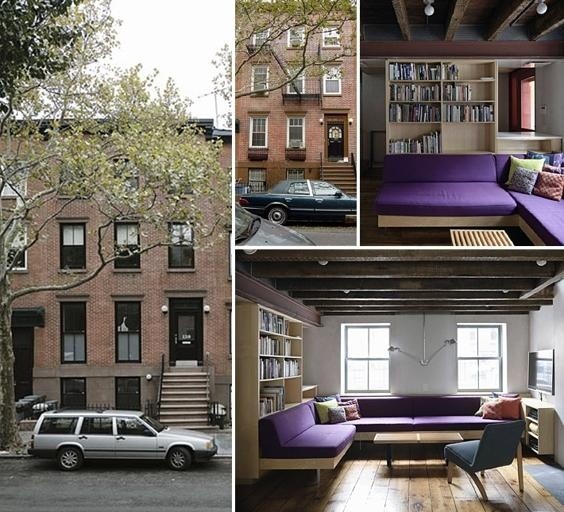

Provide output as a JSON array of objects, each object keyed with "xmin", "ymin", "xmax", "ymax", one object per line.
[{"xmin": 528, "ymin": 349, "xmax": 554, "ymax": 396}]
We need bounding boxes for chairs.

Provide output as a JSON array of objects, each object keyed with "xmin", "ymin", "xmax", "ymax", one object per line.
[{"xmin": 444, "ymin": 421, "xmax": 527, "ymax": 503}]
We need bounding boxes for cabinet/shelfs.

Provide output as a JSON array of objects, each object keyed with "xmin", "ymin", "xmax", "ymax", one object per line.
[
  {"xmin": 522, "ymin": 395, "xmax": 556, "ymax": 456},
  {"xmin": 235, "ymin": 301, "xmax": 285, "ymax": 488},
  {"xmin": 438, "ymin": 56, "xmax": 501, "ymax": 154},
  {"xmin": 301, "ymin": 385, "xmax": 318, "ymax": 403},
  {"xmin": 283, "ymin": 313, "xmax": 304, "ymax": 411},
  {"xmin": 382, "ymin": 56, "xmax": 443, "ymax": 154},
  {"xmin": 492, "ymin": 133, "xmax": 564, "ymax": 154}
]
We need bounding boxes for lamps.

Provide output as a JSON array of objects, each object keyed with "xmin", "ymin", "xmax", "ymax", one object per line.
[
  {"xmin": 319, "ymin": 118, "xmax": 325, "ymax": 125},
  {"xmin": 426, "ymin": 0, "xmax": 436, "ymax": 16},
  {"xmin": 386, "ymin": 337, "xmax": 458, "ymax": 367},
  {"xmin": 145, "ymin": 373, "xmax": 152, "ymax": 382},
  {"xmin": 161, "ymin": 304, "xmax": 168, "ymax": 317},
  {"xmin": 202, "ymin": 304, "xmax": 210, "ymax": 314},
  {"xmin": 349, "ymin": 118, "xmax": 353, "ymax": 126},
  {"xmin": 536, "ymin": 0, "xmax": 550, "ymax": 14}
]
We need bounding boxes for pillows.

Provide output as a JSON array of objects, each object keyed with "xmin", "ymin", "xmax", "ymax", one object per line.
[
  {"xmin": 504, "ymin": 150, "xmax": 563, "ymax": 203},
  {"xmin": 474, "ymin": 391, "xmax": 522, "ymax": 421},
  {"xmin": 312, "ymin": 392, "xmax": 363, "ymax": 426}
]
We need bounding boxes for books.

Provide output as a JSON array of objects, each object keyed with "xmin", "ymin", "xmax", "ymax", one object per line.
[
  {"xmin": 389, "ymin": 62, "xmax": 494, "ymax": 155},
  {"xmin": 258, "ymin": 308, "xmax": 299, "ymax": 415}
]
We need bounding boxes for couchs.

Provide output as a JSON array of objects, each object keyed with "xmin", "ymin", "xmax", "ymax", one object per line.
[
  {"xmin": 374, "ymin": 149, "xmax": 564, "ymax": 245},
  {"xmin": 259, "ymin": 395, "xmax": 530, "ymax": 500}
]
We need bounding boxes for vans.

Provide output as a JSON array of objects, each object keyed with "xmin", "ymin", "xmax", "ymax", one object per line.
[{"xmin": 26, "ymin": 407, "xmax": 218, "ymax": 470}]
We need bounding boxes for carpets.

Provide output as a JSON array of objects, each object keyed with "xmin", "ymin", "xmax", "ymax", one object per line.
[{"xmin": 527, "ymin": 463, "xmax": 564, "ymax": 508}]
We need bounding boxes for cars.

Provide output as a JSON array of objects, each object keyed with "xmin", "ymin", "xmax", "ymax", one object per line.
[
  {"xmin": 235, "ymin": 202, "xmax": 317, "ymax": 246},
  {"xmin": 240, "ymin": 178, "xmax": 356, "ymax": 227}
]
